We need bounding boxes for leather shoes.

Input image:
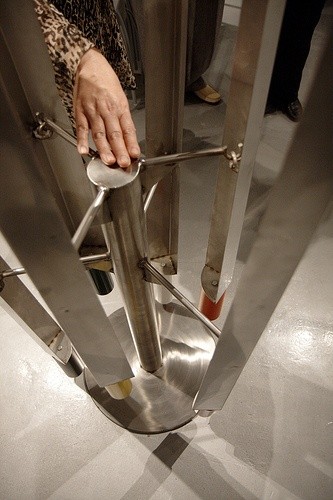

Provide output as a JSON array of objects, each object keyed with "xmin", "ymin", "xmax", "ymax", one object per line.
[{"xmin": 279, "ymin": 97, "xmax": 302, "ymax": 122}]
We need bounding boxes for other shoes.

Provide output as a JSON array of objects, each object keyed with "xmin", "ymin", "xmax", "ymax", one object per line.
[{"xmin": 196, "ymin": 85, "xmax": 222, "ymax": 104}]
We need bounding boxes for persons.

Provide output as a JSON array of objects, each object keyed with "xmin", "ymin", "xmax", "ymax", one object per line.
[
  {"xmin": 185, "ymin": 0, "xmax": 222, "ymax": 102},
  {"xmin": 32, "ymin": 0, "xmax": 141, "ymax": 168},
  {"xmin": 265, "ymin": 0, "xmax": 326, "ymax": 121}
]
